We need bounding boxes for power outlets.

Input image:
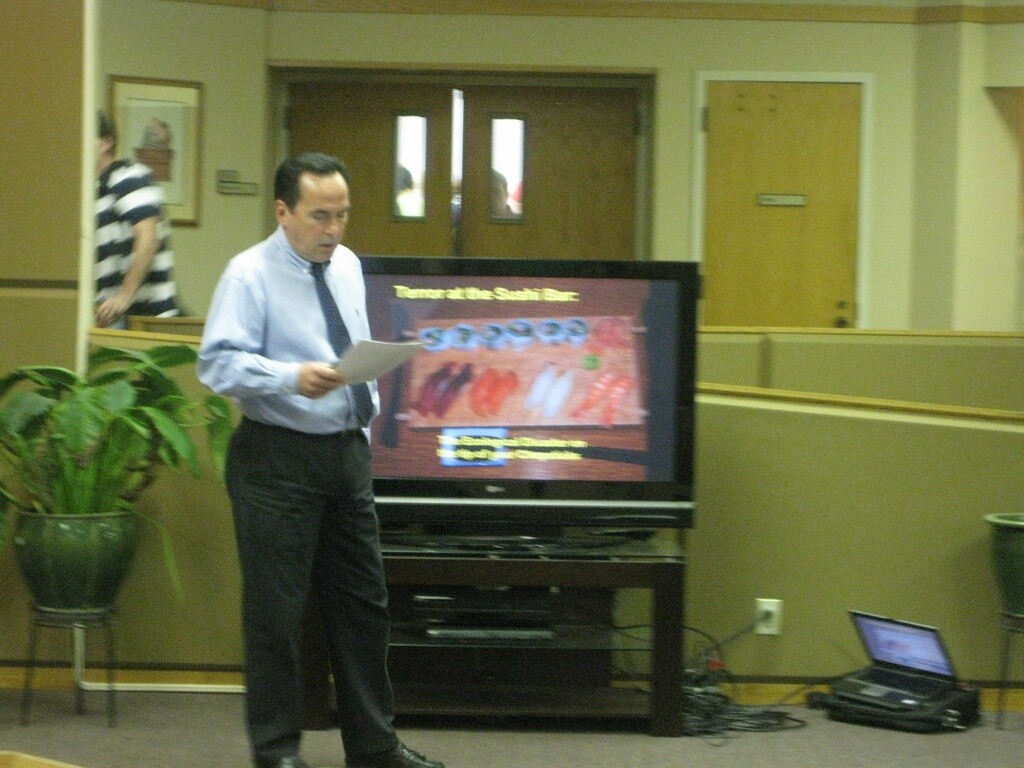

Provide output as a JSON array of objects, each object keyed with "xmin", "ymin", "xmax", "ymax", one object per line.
[{"xmin": 753, "ymin": 596, "xmax": 783, "ymax": 636}]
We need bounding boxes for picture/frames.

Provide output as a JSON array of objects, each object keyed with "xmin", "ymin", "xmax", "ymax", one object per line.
[{"xmin": 107, "ymin": 74, "xmax": 202, "ymax": 228}]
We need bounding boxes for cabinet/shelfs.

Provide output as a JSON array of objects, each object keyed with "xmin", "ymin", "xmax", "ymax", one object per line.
[{"xmin": 298, "ymin": 537, "xmax": 687, "ymax": 731}]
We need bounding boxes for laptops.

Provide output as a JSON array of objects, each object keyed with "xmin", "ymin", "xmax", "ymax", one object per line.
[{"xmin": 833, "ymin": 609, "xmax": 957, "ymax": 711}]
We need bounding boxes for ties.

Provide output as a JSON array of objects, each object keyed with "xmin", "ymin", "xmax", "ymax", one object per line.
[{"xmin": 308, "ymin": 261, "xmax": 374, "ymax": 428}]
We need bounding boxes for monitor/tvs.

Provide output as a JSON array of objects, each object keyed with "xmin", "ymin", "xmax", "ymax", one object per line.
[{"xmin": 356, "ymin": 255, "xmax": 699, "ymax": 559}]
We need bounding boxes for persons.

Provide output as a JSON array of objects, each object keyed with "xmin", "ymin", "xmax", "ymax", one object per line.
[
  {"xmin": 93, "ymin": 111, "xmax": 184, "ymax": 327},
  {"xmin": 394, "ymin": 161, "xmax": 523, "ymax": 257},
  {"xmin": 195, "ymin": 152, "xmax": 445, "ymax": 768}
]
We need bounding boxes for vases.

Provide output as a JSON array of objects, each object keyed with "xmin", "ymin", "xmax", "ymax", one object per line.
[{"xmin": 986, "ymin": 513, "xmax": 1024, "ymax": 616}]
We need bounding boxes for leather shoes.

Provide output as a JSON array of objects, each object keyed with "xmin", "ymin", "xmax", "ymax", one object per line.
[
  {"xmin": 254, "ymin": 751, "xmax": 306, "ymax": 768},
  {"xmin": 345, "ymin": 741, "xmax": 445, "ymax": 768}
]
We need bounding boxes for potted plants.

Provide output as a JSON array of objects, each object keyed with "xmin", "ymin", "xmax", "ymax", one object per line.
[{"xmin": 0, "ymin": 342, "xmax": 237, "ymax": 616}]
